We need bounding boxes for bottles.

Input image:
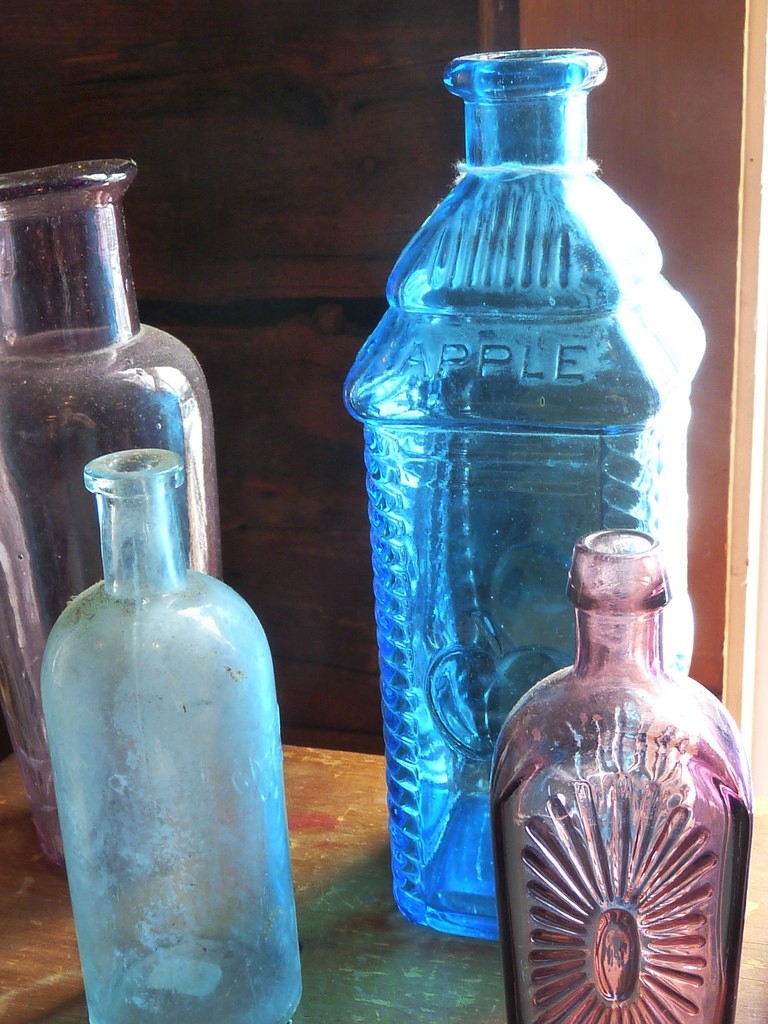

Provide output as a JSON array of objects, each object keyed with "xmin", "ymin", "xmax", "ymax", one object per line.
[
  {"xmin": 37, "ymin": 442, "xmax": 307, "ymax": 1022},
  {"xmin": 0, "ymin": 145, "xmax": 229, "ymax": 881},
  {"xmin": 480, "ymin": 524, "xmax": 757, "ymax": 1024},
  {"xmin": 342, "ymin": 40, "xmax": 709, "ymax": 948}
]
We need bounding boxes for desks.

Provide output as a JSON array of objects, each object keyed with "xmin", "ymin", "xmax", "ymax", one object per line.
[{"xmin": 0, "ymin": 738, "xmax": 768, "ymax": 1024}]
